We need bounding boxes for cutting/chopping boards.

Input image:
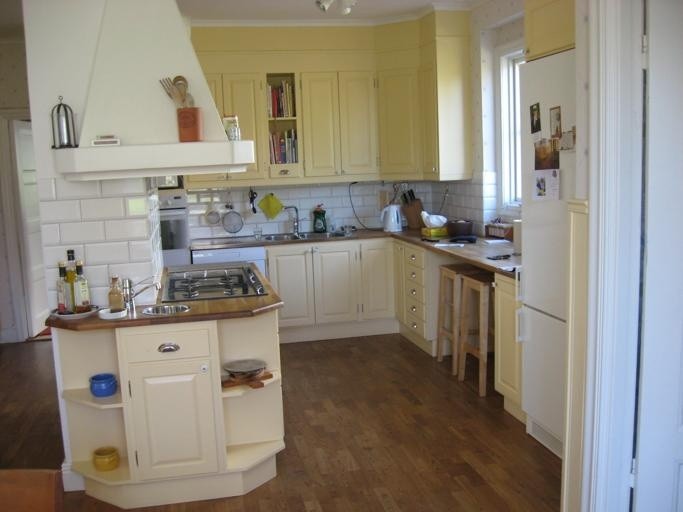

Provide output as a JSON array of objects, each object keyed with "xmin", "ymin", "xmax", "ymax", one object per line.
[{"xmin": 220, "ymin": 372, "xmax": 273, "ymax": 389}]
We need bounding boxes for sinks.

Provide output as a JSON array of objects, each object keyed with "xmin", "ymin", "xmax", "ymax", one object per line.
[
  {"xmin": 300, "ymin": 232, "xmax": 345, "ymax": 239},
  {"xmin": 142, "ymin": 304, "xmax": 191, "ymax": 315},
  {"xmin": 265, "ymin": 234, "xmax": 299, "ymax": 241}
]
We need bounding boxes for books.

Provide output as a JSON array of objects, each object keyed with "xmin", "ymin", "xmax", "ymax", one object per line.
[
  {"xmin": 266, "ymin": 80, "xmax": 294, "ymax": 118},
  {"xmin": 269, "ymin": 128, "xmax": 298, "ymax": 164}
]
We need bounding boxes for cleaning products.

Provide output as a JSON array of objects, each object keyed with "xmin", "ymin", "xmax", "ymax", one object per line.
[{"xmin": 311, "ymin": 204, "xmax": 327, "ymax": 233}]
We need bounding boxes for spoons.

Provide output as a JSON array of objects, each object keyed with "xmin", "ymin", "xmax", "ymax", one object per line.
[{"xmin": 173, "ymin": 76, "xmax": 189, "ymax": 108}]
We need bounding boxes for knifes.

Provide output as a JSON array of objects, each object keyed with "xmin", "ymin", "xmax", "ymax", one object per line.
[{"xmin": 402, "ymin": 189, "xmax": 415, "ymax": 206}]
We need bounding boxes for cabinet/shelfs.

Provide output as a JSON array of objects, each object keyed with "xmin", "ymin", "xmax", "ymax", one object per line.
[
  {"xmin": 181, "ymin": 71, "xmax": 267, "ymax": 190},
  {"xmin": 357, "ymin": 236, "xmax": 394, "ymax": 322},
  {"xmin": 522, "ymin": 0, "xmax": 575, "ymax": 63},
  {"xmin": 392, "ymin": 238, "xmax": 494, "ymax": 358},
  {"xmin": 44, "ymin": 300, "xmax": 287, "ymax": 511},
  {"xmin": 419, "ymin": 58, "xmax": 473, "ymax": 183},
  {"xmin": 371, "ymin": 64, "xmax": 423, "ymax": 181},
  {"xmin": 263, "ymin": 70, "xmax": 305, "ymax": 190},
  {"xmin": 266, "ymin": 240, "xmax": 358, "ymax": 331},
  {"xmin": 301, "ymin": 67, "xmax": 379, "ymax": 183},
  {"xmin": 492, "ymin": 271, "xmax": 527, "ymax": 427}
]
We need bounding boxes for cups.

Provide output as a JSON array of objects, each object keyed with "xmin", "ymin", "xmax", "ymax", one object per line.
[
  {"xmin": 226, "ymin": 128, "xmax": 241, "ymax": 140},
  {"xmin": 176, "ymin": 107, "xmax": 203, "ymax": 144},
  {"xmin": 343, "ymin": 222, "xmax": 352, "ymax": 236},
  {"xmin": 448, "ymin": 219, "xmax": 473, "ymax": 237},
  {"xmin": 253, "ymin": 227, "xmax": 262, "ymax": 240}
]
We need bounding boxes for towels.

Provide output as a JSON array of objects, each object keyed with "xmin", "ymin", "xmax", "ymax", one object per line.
[{"xmin": 257, "ymin": 193, "xmax": 284, "ymax": 220}]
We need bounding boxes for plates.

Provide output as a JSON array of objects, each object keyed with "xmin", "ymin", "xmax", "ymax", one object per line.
[{"xmin": 51, "ymin": 304, "xmax": 100, "ymax": 319}]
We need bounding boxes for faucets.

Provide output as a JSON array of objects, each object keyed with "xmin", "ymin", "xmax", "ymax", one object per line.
[
  {"xmin": 122, "ymin": 277, "xmax": 162, "ymax": 310},
  {"xmin": 281, "ymin": 205, "xmax": 299, "ymax": 234}
]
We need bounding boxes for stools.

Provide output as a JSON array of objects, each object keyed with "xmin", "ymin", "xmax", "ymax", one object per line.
[
  {"xmin": 434, "ymin": 259, "xmax": 489, "ymax": 379},
  {"xmin": 456, "ymin": 270, "xmax": 495, "ymax": 397}
]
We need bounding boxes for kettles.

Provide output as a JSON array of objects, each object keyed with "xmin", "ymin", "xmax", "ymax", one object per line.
[{"xmin": 380, "ymin": 203, "xmax": 403, "ymax": 232}]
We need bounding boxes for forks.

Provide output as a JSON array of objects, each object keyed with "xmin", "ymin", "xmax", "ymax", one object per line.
[{"xmin": 157, "ymin": 77, "xmax": 183, "ymax": 106}]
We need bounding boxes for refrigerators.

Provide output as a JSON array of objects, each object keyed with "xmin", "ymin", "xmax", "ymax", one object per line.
[{"xmin": 513, "ymin": 44, "xmax": 575, "ymax": 462}]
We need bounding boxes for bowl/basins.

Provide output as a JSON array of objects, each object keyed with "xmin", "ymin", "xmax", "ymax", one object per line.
[{"xmin": 223, "ymin": 359, "xmax": 268, "ymax": 378}]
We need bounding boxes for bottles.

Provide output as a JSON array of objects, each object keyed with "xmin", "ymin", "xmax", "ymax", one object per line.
[
  {"xmin": 93, "ymin": 446, "xmax": 120, "ymax": 472},
  {"xmin": 56, "ymin": 260, "xmax": 73, "ymax": 315},
  {"xmin": 486, "ymin": 223, "xmax": 505, "ymax": 239},
  {"xmin": 108, "ymin": 275, "xmax": 125, "ymax": 313},
  {"xmin": 88, "ymin": 372, "xmax": 118, "ymax": 396},
  {"xmin": 222, "ymin": 113, "xmax": 241, "ymax": 141},
  {"xmin": 65, "ymin": 249, "xmax": 77, "ymax": 313},
  {"xmin": 73, "ymin": 260, "xmax": 92, "ymax": 313}
]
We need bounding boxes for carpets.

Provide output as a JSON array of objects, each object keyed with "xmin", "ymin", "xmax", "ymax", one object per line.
[{"xmin": 0, "ymin": 467, "xmax": 64, "ymax": 512}]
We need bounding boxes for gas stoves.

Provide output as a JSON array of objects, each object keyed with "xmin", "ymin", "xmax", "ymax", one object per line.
[{"xmin": 160, "ymin": 266, "xmax": 269, "ymax": 304}]
